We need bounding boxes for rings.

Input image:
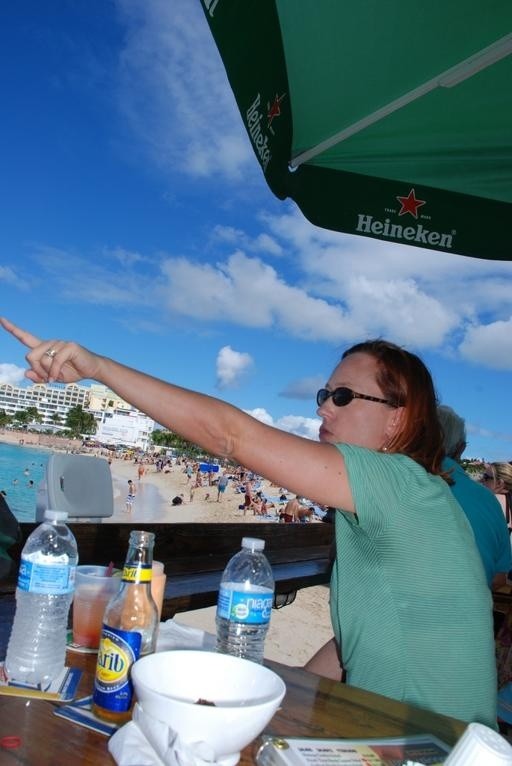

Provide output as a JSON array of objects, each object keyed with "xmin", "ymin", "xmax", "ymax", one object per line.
[{"xmin": 44, "ymin": 347, "xmax": 57, "ymax": 359}]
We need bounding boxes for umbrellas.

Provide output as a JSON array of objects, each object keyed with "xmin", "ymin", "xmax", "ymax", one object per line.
[{"xmin": 198, "ymin": 0, "xmax": 512, "ymax": 261}]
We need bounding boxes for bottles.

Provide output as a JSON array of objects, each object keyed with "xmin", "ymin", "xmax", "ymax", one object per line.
[
  {"xmin": 93, "ymin": 529, "xmax": 159, "ymax": 723},
  {"xmin": 2, "ymin": 510, "xmax": 79, "ymax": 685},
  {"xmin": 215, "ymin": 536, "xmax": 275, "ymax": 666}
]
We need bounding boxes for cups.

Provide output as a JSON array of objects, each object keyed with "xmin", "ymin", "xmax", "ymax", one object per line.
[{"xmin": 72, "ymin": 564, "xmax": 125, "ymax": 649}]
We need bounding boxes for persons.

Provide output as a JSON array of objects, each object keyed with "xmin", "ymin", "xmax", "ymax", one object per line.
[
  {"xmin": 432, "ymin": 403, "xmax": 512, "ymax": 639},
  {"xmin": 0, "ymin": 317, "xmax": 501, "ymax": 732},
  {"xmin": 1, "ymin": 415, "xmax": 327, "ymax": 524},
  {"xmin": 479, "ymin": 461, "xmax": 512, "ymax": 532}
]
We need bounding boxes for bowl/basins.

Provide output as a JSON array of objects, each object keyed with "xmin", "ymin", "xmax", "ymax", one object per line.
[{"xmin": 127, "ymin": 649, "xmax": 286, "ymax": 762}]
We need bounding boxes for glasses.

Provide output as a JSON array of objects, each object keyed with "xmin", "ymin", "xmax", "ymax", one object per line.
[{"xmin": 314, "ymin": 386, "xmax": 407, "ymax": 412}]
